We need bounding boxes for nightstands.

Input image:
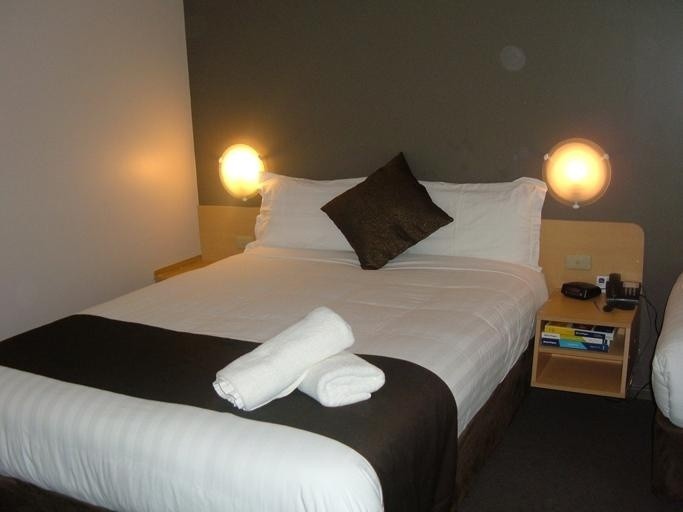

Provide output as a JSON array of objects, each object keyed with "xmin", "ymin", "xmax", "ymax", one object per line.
[{"xmin": 154, "ymin": 255, "xmax": 208, "ymax": 283}]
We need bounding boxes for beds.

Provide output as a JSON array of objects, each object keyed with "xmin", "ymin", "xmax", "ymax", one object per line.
[
  {"xmin": 0, "ymin": 241, "xmax": 550, "ymax": 512},
  {"xmin": 650, "ymin": 271, "xmax": 683, "ymax": 504},
  {"xmin": 529, "ymin": 288, "xmax": 640, "ymax": 400}
]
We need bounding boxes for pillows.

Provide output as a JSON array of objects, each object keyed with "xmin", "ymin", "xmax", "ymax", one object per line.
[{"xmin": 248, "ymin": 151, "xmax": 548, "ymax": 272}]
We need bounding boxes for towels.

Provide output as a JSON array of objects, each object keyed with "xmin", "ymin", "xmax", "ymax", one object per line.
[
  {"xmin": 296, "ymin": 351, "xmax": 385, "ymax": 407},
  {"xmin": 211, "ymin": 305, "xmax": 356, "ymax": 413}
]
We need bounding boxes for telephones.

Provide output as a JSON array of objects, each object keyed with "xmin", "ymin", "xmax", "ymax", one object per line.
[{"xmin": 606, "ymin": 273, "xmax": 641, "ymax": 304}]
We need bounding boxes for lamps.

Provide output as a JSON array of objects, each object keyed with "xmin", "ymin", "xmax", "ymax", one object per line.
[
  {"xmin": 541, "ymin": 138, "xmax": 612, "ymax": 209},
  {"xmin": 218, "ymin": 143, "xmax": 267, "ymax": 202}
]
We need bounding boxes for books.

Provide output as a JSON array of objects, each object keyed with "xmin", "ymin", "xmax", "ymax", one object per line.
[{"xmin": 540, "ymin": 321, "xmax": 616, "ymax": 352}]
